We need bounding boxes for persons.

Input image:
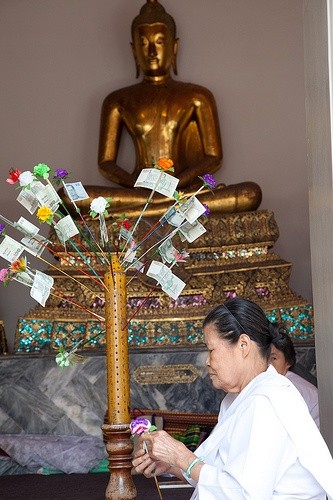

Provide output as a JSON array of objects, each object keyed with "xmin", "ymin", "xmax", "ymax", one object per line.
[
  {"xmin": 219, "ymin": 328, "xmax": 321, "ymax": 429},
  {"xmin": 131, "ymin": 302, "xmax": 333, "ymax": 500},
  {"xmin": 53, "ymin": 0, "xmax": 261, "ymax": 221}
]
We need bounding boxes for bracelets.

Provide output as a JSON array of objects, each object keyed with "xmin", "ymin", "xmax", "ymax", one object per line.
[{"xmin": 186, "ymin": 456, "xmax": 204, "ymax": 478}]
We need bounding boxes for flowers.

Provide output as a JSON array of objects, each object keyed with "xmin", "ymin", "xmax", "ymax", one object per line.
[
  {"xmin": 0, "ymin": 163, "xmax": 217, "ymax": 331},
  {"xmin": 54, "ymin": 331, "xmax": 105, "ymax": 368},
  {"xmin": 129, "ymin": 418, "xmax": 164, "ymax": 500}
]
